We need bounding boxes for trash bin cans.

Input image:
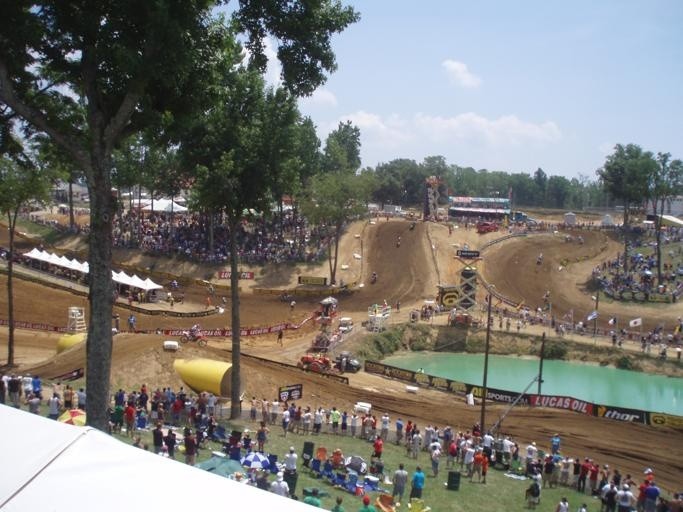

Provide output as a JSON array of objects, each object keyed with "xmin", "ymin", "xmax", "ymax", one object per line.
[
  {"xmin": 301, "ymin": 442, "xmax": 314, "ymax": 459},
  {"xmin": 447, "ymin": 472, "xmax": 460, "ymax": 490}
]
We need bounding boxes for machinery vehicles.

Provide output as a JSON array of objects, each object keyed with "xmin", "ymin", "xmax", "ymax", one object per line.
[
  {"xmin": 331, "ymin": 350, "xmax": 362, "ymax": 374},
  {"xmin": 448, "ymin": 312, "xmax": 478, "ymax": 330},
  {"xmin": 295, "ymin": 345, "xmax": 335, "ymax": 375}
]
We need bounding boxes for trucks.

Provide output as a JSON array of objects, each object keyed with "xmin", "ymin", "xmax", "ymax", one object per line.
[{"xmin": 361, "ymin": 203, "xmax": 419, "ymax": 222}]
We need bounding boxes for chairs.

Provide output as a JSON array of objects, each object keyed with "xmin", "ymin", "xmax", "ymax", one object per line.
[{"xmin": 301, "ymin": 453, "xmax": 359, "ymax": 494}]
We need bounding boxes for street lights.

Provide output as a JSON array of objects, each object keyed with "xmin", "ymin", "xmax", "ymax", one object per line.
[{"xmin": 489, "ymin": 191, "xmax": 499, "ymax": 221}]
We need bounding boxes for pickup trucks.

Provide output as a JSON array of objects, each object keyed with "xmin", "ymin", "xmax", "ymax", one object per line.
[{"xmin": 476, "ymin": 222, "xmax": 500, "ymax": 234}]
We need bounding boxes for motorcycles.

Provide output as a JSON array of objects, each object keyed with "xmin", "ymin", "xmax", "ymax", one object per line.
[
  {"xmin": 179, "ymin": 331, "xmax": 209, "ymax": 348},
  {"xmin": 370, "ymin": 273, "xmax": 379, "ymax": 284}
]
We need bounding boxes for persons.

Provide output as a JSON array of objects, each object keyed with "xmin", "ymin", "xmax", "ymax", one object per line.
[
  {"xmin": 2, "ymin": 211, "xmax": 682, "ymax": 373},
  {"xmin": 1, "ymin": 371, "xmax": 683, "ymax": 512}
]
